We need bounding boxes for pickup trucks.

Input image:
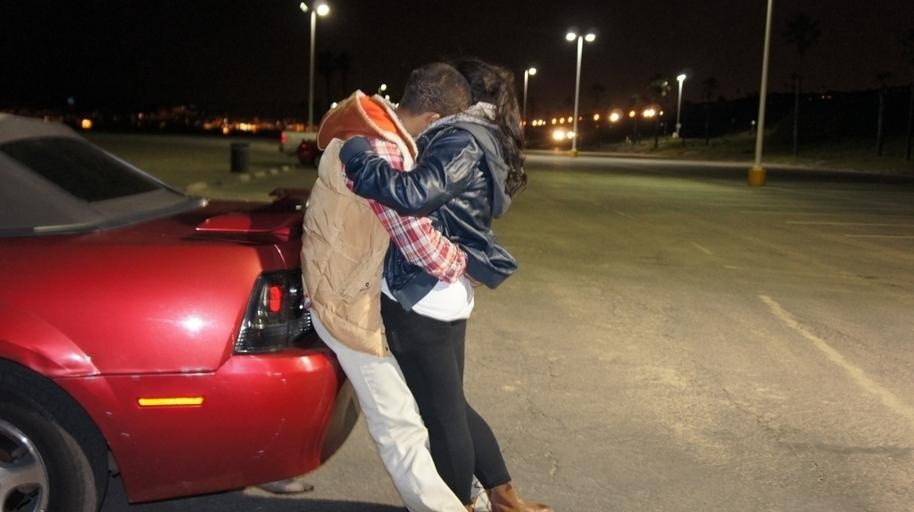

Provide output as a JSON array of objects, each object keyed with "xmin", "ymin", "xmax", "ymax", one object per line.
[{"xmin": 280, "ymin": 130, "xmax": 321, "ymax": 167}]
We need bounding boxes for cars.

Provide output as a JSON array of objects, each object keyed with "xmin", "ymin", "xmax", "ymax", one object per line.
[{"xmin": 0, "ymin": 110, "xmax": 361, "ymax": 509}]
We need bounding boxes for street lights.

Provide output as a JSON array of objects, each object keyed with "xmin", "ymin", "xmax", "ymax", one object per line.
[
  {"xmin": 674, "ymin": 73, "xmax": 688, "ymax": 136},
  {"xmin": 521, "ymin": 68, "xmax": 536, "ymax": 132},
  {"xmin": 299, "ymin": 2, "xmax": 329, "ymax": 130},
  {"xmin": 378, "ymin": 84, "xmax": 386, "ymax": 95},
  {"xmin": 564, "ymin": 31, "xmax": 597, "ymax": 158}
]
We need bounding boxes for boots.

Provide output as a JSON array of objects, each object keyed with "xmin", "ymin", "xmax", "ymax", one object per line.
[{"xmin": 489, "ymin": 479, "xmax": 552, "ymax": 512}]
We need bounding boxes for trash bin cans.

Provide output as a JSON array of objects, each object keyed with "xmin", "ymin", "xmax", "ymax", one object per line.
[{"xmin": 230, "ymin": 142, "xmax": 250, "ymax": 173}]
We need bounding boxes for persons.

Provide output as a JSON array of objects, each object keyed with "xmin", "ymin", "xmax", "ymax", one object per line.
[
  {"xmin": 299, "ymin": 60, "xmax": 471, "ymax": 512},
  {"xmin": 341, "ymin": 58, "xmax": 549, "ymax": 512}
]
